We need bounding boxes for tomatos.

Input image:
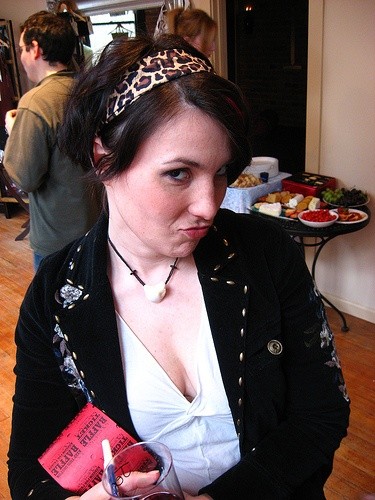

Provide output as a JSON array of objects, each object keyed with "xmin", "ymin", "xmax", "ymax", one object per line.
[{"xmin": 301, "ymin": 209, "xmax": 336, "ymax": 222}]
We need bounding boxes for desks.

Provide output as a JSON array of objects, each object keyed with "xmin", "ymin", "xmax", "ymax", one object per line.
[{"xmin": 251, "ymin": 204, "xmax": 371, "ymax": 333}]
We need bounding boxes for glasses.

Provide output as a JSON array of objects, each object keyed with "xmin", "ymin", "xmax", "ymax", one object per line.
[{"xmin": 15, "ymin": 43, "xmax": 32, "ymax": 55}]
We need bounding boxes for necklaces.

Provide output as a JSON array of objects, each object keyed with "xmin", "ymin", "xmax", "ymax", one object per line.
[{"xmin": 107, "ymin": 232, "xmax": 180, "ymax": 303}]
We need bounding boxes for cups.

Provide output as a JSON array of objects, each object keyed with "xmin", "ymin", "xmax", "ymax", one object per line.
[{"xmin": 101, "ymin": 441, "xmax": 185, "ymax": 500}]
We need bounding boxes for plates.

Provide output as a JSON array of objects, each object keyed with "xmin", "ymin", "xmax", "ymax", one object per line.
[
  {"xmin": 297, "ymin": 209, "xmax": 339, "ymax": 228},
  {"xmin": 247, "ymin": 205, "xmax": 298, "ymax": 222},
  {"xmin": 329, "ymin": 208, "xmax": 368, "ymax": 224},
  {"xmin": 323, "ymin": 196, "xmax": 370, "ymax": 208}
]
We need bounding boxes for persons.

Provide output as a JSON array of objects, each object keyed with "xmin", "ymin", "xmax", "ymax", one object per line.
[
  {"xmin": 3, "ymin": 10, "xmax": 102, "ymax": 272},
  {"xmin": 168, "ymin": 8, "xmax": 221, "ymax": 60},
  {"xmin": 5, "ymin": 35, "xmax": 350, "ymax": 500}
]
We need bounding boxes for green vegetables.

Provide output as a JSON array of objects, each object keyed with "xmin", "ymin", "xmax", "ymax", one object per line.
[{"xmin": 322, "ymin": 187, "xmax": 368, "ymax": 207}]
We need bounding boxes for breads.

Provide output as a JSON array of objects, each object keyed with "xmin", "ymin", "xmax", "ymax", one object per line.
[
  {"xmin": 229, "ymin": 175, "xmax": 264, "ymax": 187},
  {"xmin": 255, "ymin": 191, "xmax": 320, "ymax": 219}
]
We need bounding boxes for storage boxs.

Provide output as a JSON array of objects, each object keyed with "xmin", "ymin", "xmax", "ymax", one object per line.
[{"xmin": 220, "ymin": 172, "xmax": 292, "ymax": 214}]
